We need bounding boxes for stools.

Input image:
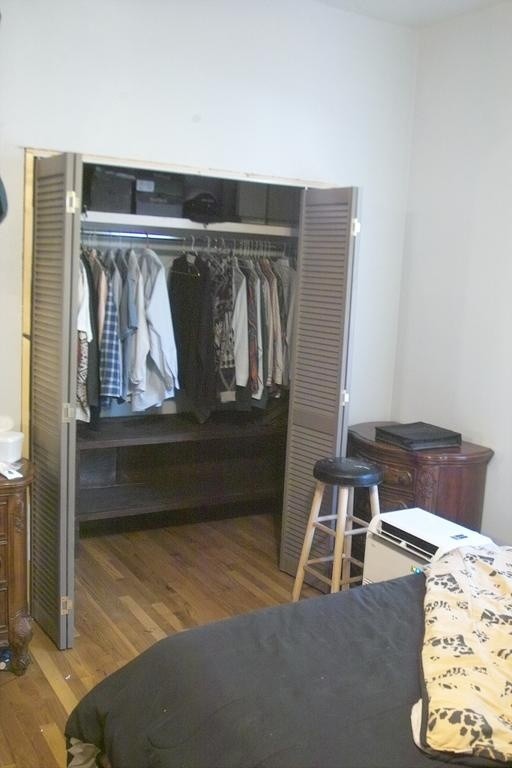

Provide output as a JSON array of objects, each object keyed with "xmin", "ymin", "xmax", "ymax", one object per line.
[{"xmin": 293, "ymin": 450, "xmax": 382, "ymax": 605}]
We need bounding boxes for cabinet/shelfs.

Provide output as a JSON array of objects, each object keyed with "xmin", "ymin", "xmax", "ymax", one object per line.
[
  {"xmin": 76, "ymin": 209, "xmax": 302, "ymax": 529},
  {"xmin": 1, "ymin": 454, "xmax": 42, "ymax": 681},
  {"xmin": 344, "ymin": 418, "xmax": 494, "ymax": 589}
]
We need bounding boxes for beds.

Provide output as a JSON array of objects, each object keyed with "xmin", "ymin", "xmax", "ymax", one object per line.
[{"xmin": 60, "ymin": 533, "xmax": 510, "ymax": 765}]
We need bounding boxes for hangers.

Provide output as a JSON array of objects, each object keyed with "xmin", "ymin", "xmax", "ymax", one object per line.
[{"xmin": 77, "ymin": 227, "xmax": 297, "ymax": 270}]
